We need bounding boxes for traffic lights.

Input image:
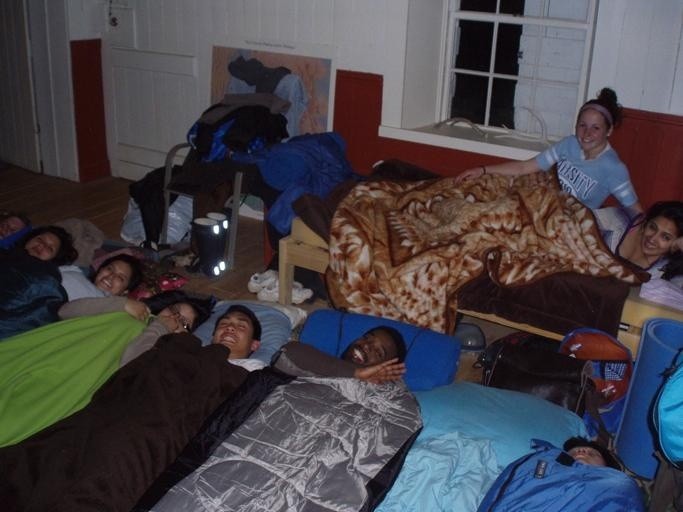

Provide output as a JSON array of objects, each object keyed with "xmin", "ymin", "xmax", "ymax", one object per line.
[
  {"xmin": 195, "ymin": 301, "xmax": 289, "ymax": 362},
  {"xmin": 409, "ymin": 377, "xmax": 591, "ymax": 465},
  {"xmin": 301, "ymin": 308, "xmax": 462, "ymax": 392}
]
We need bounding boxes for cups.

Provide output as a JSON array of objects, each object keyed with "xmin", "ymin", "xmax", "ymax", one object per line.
[{"xmin": 168, "ymin": 304, "xmax": 191, "ymax": 333}]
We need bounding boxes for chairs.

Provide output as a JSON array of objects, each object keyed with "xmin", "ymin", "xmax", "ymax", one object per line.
[
  {"xmin": 246, "ymin": 269, "xmax": 302, "ymax": 291},
  {"xmin": 257, "ymin": 285, "xmax": 313, "ymax": 305}
]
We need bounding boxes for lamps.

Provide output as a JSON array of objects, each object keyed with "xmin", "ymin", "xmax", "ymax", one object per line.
[{"xmin": 161, "ymin": 72, "xmax": 299, "ymax": 272}]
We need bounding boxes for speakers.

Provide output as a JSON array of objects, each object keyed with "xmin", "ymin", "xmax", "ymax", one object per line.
[
  {"xmin": 206, "ymin": 211, "xmax": 226, "ymax": 273},
  {"xmin": 184, "ymin": 217, "xmax": 219, "ymax": 278}
]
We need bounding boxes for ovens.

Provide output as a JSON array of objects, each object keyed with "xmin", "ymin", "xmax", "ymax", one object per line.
[{"xmin": 277, "ymin": 213, "xmax": 682, "ymax": 378}]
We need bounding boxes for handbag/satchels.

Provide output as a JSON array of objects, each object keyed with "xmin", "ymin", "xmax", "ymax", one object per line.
[{"xmin": 480, "ymin": 165, "xmax": 486, "ymax": 175}]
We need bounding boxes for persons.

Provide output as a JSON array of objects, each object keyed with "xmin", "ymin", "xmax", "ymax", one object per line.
[
  {"xmin": 57, "ymin": 290, "xmax": 210, "ymax": 370},
  {"xmin": 0, "ymin": 212, "xmax": 31, "ymax": 249},
  {"xmin": 211, "ymin": 304, "xmax": 267, "ymax": 371},
  {"xmin": 563, "ymin": 436, "xmax": 622, "ymax": 471},
  {"xmin": 78, "ymin": 254, "xmax": 143, "ymax": 296},
  {"xmin": 271, "ymin": 326, "xmax": 408, "ymax": 385},
  {"xmin": 23, "ymin": 224, "xmax": 71, "ymax": 262},
  {"xmin": 591, "ymin": 199, "xmax": 683, "ymax": 290},
  {"xmin": 453, "ymin": 87, "xmax": 645, "ymax": 226}
]
